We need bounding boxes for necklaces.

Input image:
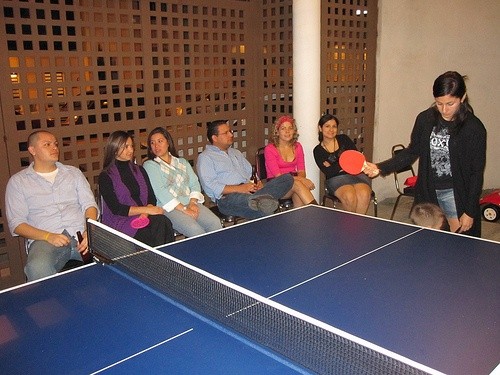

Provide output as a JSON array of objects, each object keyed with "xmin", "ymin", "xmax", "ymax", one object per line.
[{"xmin": 323, "ymin": 138, "xmax": 335, "ymax": 153}]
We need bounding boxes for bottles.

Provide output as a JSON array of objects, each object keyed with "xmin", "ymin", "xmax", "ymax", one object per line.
[
  {"xmin": 76, "ymin": 231, "xmax": 94, "ymax": 265},
  {"xmin": 249, "ymin": 165, "xmax": 258, "ymax": 194}
]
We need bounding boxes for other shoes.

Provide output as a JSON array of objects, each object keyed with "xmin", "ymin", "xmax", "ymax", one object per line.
[{"xmin": 247, "ymin": 195, "xmax": 279, "ymax": 213}]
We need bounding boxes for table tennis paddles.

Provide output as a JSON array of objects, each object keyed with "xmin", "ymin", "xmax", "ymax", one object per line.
[{"xmin": 339, "ymin": 150, "xmax": 380, "ymax": 175}]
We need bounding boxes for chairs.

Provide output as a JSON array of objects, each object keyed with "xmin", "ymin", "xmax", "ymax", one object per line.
[
  {"xmin": 321, "ymin": 143, "xmax": 421, "ymax": 221},
  {"xmin": 254, "ymin": 144, "xmax": 295, "ymax": 211},
  {"xmin": 189, "ymin": 152, "xmax": 246, "ymax": 228}
]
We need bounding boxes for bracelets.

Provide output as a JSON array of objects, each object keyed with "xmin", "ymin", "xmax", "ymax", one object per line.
[{"xmin": 45, "ymin": 233, "xmax": 50, "ymax": 241}]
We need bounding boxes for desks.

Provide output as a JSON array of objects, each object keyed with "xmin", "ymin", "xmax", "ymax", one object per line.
[{"xmin": 0, "ymin": 204, "xmax": 500, "ymax": 375}]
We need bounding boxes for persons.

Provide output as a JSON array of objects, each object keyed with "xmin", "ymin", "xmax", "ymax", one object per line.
[
  {"xmin": 196, "ymin": 120, "xmax": 294, "ymax": 220},
  {"xmin": 142, "ymin": 127, "xmax": 223, "ymax": 237},
  {"xmin": 363, "ymin": 71, "xmax": 487, "ymax": 238},
  {"xmin": 265, "ymin": 115, "xmax": 319, "ymax": 208},
  {"xmin": 5, "ymin": 130, "xmax": 100, "ymax": 283},
  {"xmin": 98, "ymin": 130, "xmax": 175, "ymax": 251},
  {"xmin": 313, "ymin": 114, "xmax": 372, "ymax": 214}
]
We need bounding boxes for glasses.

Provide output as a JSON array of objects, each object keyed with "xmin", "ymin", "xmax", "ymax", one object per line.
[{"xmin": 216, "ymin": 129, "xmax": 234, "ymax": 135}]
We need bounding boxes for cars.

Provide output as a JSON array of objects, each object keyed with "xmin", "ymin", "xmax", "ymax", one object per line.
[{"xmin": 479, "ymin": 187, "xmax": 500, "ymax": 222}]
